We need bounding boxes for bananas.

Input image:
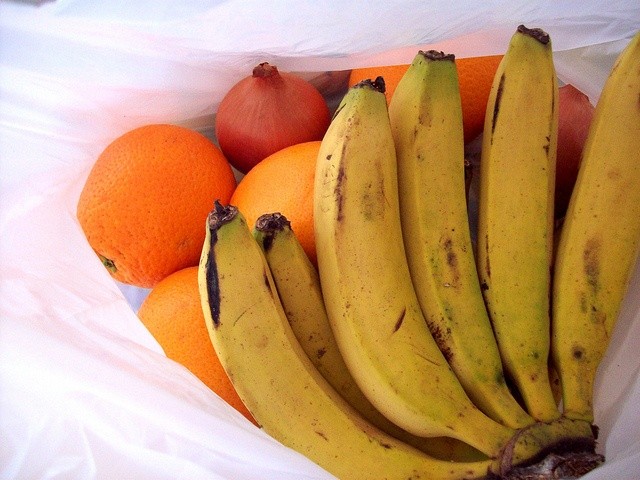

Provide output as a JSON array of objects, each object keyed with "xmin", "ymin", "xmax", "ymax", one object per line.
[{"xmin": 197, "ymin": 22, "xmax": 639, "ymax": 479}]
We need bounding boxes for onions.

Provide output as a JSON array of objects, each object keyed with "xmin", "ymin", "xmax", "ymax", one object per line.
[
  {"xmin": 217, "ymin": 63, "xmax": 328, "ymax": 172},
  {"xmin": 555, "ymin": 84, "xmax": 596, "ymax": 163}
]
